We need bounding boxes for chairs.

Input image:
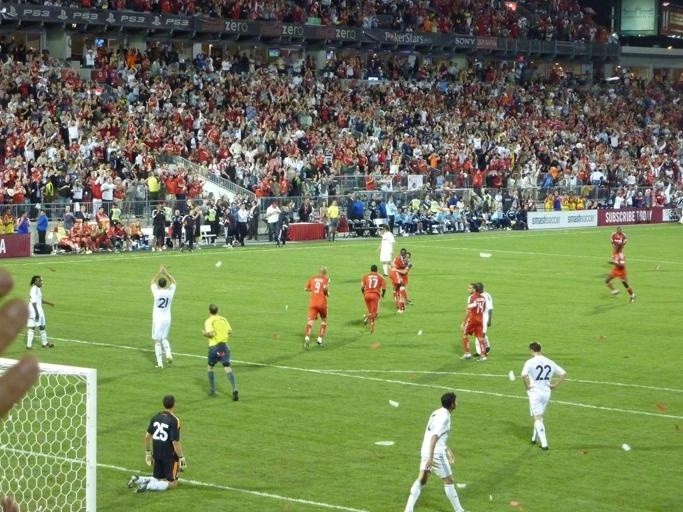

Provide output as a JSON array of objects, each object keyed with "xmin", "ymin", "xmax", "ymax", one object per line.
[{"xmin": 345, "ymin": 218, "xmax": 383, "ymax": 238}]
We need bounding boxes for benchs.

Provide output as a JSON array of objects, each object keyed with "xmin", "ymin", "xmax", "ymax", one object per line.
[{"xmin": 139, "ymin": 224, "xmax": 217, "ymax": 246}]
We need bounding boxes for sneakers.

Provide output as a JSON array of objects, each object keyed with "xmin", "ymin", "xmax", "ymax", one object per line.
[
  {"xmin": 232, "ymin": 390, "xmax": 238, "ymax": 401},
  {"xmin": 41, "ymin": 342, "xmax": 54, "ymax": 348},
  {"xmin": 459, "ymin": 354, "xmax": 471, "ymax": 360},
  {"xmin": 531, "ymin": 435, "xmax": 537, "ymax": 446},
  {"xmin": 127, "ymin": 474, "xmax": 138, "ymax": 488},
  {"xmin": 303, "ymin": 336, "xmax": 312, "ymax": 353},
  {"xmin": 135, "ymin": 479, "xmax": 149, "ymax": 492},
  {"xmin": 539, "ymin": 444, "xmax": 548, "ymax": 451},
  {"xmin": 208, "ymin": 385, "xmax": 217, "ymax": 396},
  {"xmin": 609, "ymin": 289, "xmax": 637, "ymax": 303},
  {"xmin": 472, "ymin": 345, "xmax": 491, "ymax": 362},
  {"xmin": 26, "ymin": 346, "xmax": 36, "ymax": 351},
  {"xmin": 316, "ymin": 338, "xmax": 325, "ymax": 349},
  {"xmin": 363, "ymin": 314, "xmax": 376, "ymax": 335},
  {"xmin": 152, "ymin": 362, "xmax": 164, "ymax": 369},
  {"xmin": 394, "ymin": 299, "xmax": 414, "ymax": 314},
  {"xmin": 165, "ymin": 357, "xmax": 174, "ymax": 368}
]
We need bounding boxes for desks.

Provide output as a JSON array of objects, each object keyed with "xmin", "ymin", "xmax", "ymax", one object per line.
[{"xmin": 285, "ymin": 222, "xmax": 325, "ymax": 241}]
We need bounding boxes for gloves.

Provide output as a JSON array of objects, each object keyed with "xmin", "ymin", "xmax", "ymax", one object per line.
[
  {"xmin": 144, "ymin": 449, "xmax": 153, "ymax": 466},
  {"xmin": 177, "ymin": 455, "xmax": 186, "ymax": 472}
]
